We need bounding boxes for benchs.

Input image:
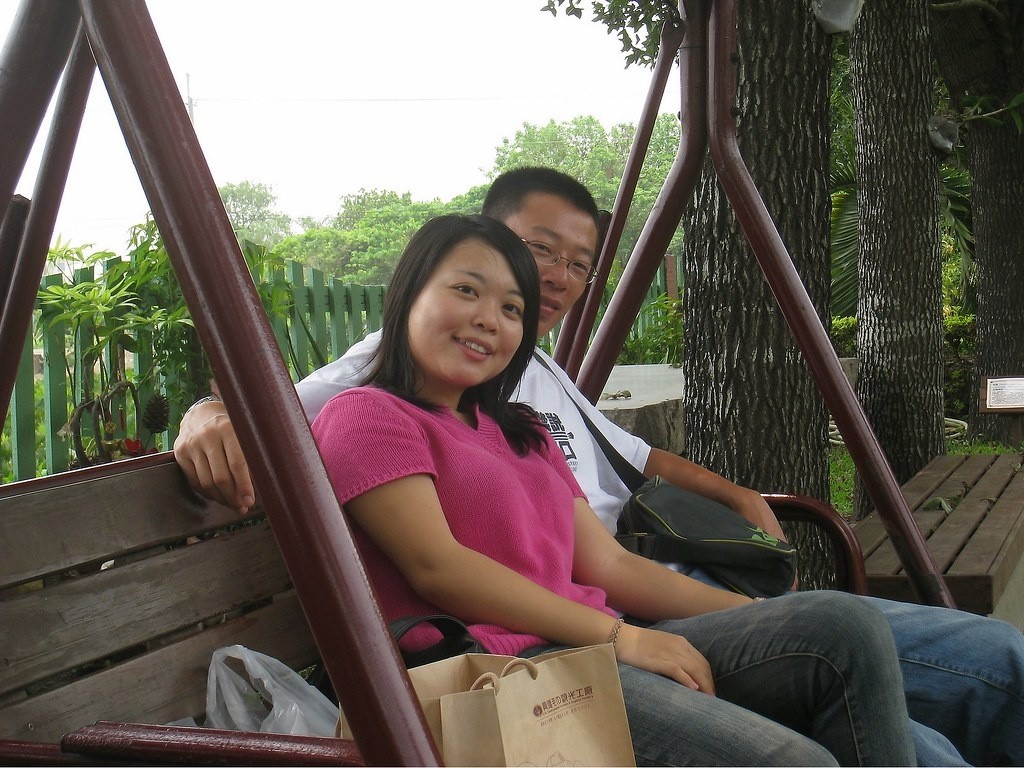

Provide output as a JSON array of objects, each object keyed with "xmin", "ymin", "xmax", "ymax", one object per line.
[
  {"xmin": 0, "ymin": 445, "xmax": 997, "ymax": 768},
  {"xmin": 849, "ymin": 455, "xmax": 1024, "ymax": 623}
]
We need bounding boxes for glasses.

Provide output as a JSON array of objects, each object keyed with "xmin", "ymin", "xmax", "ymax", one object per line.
[{"xmin": 521, "ymin": 238, "xmax": 602, "ymax": 284}]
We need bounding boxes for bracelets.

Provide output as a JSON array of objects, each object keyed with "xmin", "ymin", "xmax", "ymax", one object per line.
[
  {"xmin": 607, "ymin": 618, "xmax": 624, "ymax": 646},
  {"xmin": 184, "ymin": 395, "xmax": 223, "ymax": 416}
]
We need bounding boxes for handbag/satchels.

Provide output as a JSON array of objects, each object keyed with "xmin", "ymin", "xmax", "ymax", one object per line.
[
  {"xmin": 200, "ymin": 644, "xmax": 340, "ymax": 737},
  {"xmin": 619, "ymin": 474, "xmax": 798, "ymax": 601},
  {"xmin": 305, "ymin": 614, "xmax": 492, "ymax": 707},
  {"xmin": 335, "ymin": 643, "xmax": 637, "ymax": 767}
]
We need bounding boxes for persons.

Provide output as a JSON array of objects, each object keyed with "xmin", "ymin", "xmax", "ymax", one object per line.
[
  {"xmin": 172, "ymin": 166, "xmax": 1024, "ymax": 767},
  {"xmin": 309, "ymin": 212, "xmax": 919, "ymax": 767}
]
